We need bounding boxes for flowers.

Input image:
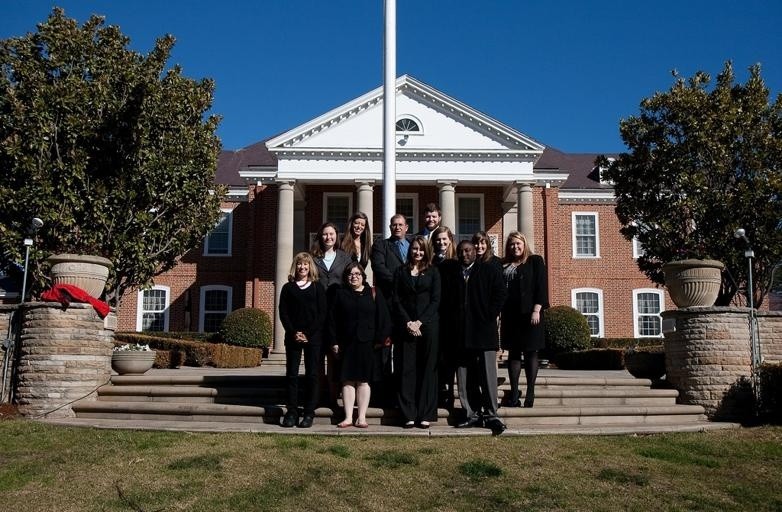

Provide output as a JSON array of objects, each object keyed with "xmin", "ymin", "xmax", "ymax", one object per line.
[
  {"xmin": 620, "ymin": 344, "xmax": 666, "ymax": 368},
  {"xmin": 113, "ymin": 343, "xmax": 153, "ymax": 352}
]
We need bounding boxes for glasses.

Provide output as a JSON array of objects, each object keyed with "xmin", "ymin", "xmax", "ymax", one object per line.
[{"xmin": 347, "ymin": 273, "xmax": 362, "ymax": 277}]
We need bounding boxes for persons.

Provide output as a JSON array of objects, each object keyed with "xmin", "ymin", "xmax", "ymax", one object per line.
[
  {"xmin": 442, "ymin": 240, "xmax": 509, "ymax": 432},
  {"xmin": 280, "ymin": 253, "xmax": 326, "ymax": 427},
  {"xmin": 471, "ymin": 232, "xmax": 504, "ymax": 380},
  {"xmin": 499, "ymin": 232, "xmax": 549, "ymax": 408},
  {"xmin": 389, "ymin": 235, "xmax": 442, "ymax": 428},
  {"xmin": 417, "ymin": 202, "xmax": 457, "ymax": 264},
  {"xmin": 309, "ymin": 221, "xmax": 353, "ymax": 409},
  {"xmin": 370, "ymin": 214, "xmax": 412, "ymax": 310},
  {"xmin": 328, "ymin": 262, "xmax": 391, "ymax": 428},
  {"xmin": 338, "ymin": 211, "xmax": 374, "ymax": 285}
]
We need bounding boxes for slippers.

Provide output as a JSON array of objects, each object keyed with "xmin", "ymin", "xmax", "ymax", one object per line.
[{"xmin": 338, "ymin": 419, "xmax": 368, "ymax": 427}]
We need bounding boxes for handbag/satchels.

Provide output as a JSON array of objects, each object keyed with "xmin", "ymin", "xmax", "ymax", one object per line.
[{"xmin": 376, "ymin": 335, "xmax": 391, "ymax": 348}]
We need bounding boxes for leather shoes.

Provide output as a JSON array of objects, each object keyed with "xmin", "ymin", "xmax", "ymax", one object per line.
[
  {"xmin": 282, "ymin": 414, "xmax": 313, "ymax": 427},
  {"xmin": 404, "ymin": 420, "xmax": 429, "ymax": 428},
  {"xmin": 458, "ymin": 418, "xmax": 480, "ymax": 427},
  {"xmin": 506, "ymin": 394, "xmax": 534, "ymax": 407}
]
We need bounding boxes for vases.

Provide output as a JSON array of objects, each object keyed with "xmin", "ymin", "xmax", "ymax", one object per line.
[
  {"xmin": 46, "ymin": 254, "xmax": 114, "ymax": 299},
  {"xmin": 111, "ymin": 350, "xmax": 157, "ymax": 375},
  {"xmin": 624, "ymin": 352, "xmax": 668, "ymax": 378},
  {"xmin": 664, "ymin": 258, "xmax": 725, "ymax": 308}
]
